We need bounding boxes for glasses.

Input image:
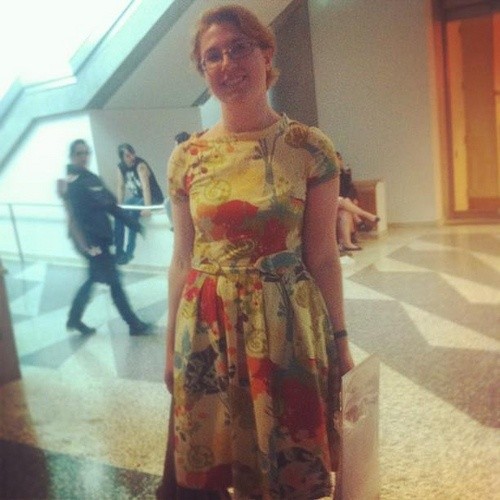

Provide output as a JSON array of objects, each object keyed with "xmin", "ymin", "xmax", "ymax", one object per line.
[{"xmin": 192, "ymin": 38, "xmax": 264, "ymax": 68}]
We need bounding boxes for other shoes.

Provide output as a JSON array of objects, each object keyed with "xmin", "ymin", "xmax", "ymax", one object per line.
[{"xmin": 63, "ymin": 316, "xmax": 160, "ymax": 337}]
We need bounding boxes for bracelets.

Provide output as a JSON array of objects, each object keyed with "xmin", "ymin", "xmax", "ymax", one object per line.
[{"xmin": 334, "ymin": 329, "xmax": 347, "ymax": 339}]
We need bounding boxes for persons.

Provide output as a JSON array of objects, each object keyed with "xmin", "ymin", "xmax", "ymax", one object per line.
[
  {"xmin": 336, "ymin": 150, "xmax": 380, "ymax": 251},
  {"xmin": 159, "ymin": 6, "xmax": 355, "ymax": 500},
  {"xmin": 165, "ymin": 132, "xmax": 191, "ymax": 232},
  {"xmin": 113, "ymin": 143, "xmax": 164, "ymax": 265},
  {"xmin": 57, "ymin": 139, "xmax": 153, "ymax": 335}
]
